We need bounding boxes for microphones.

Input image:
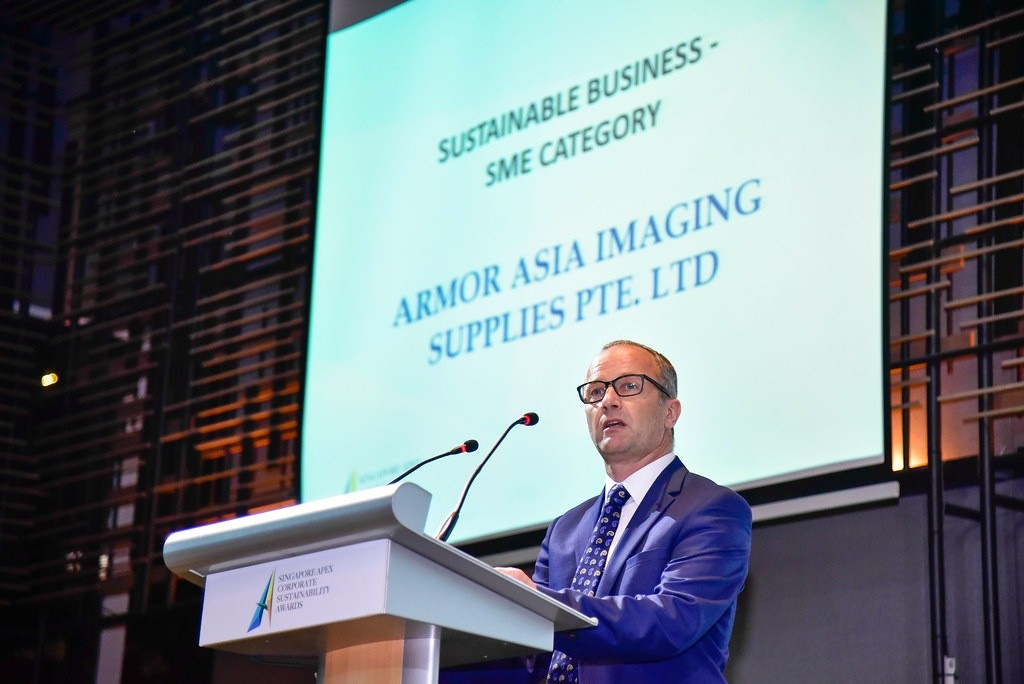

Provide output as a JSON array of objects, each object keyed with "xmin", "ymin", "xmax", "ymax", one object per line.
[
  {"xmin": 436, "ymin": 413, "xmax": 540, "ymax": 542},
  {"xmin": 386, "ymin": 440, "xmax": 478, "ymax": 483}
]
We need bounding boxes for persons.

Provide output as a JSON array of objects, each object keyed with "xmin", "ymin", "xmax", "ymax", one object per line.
[{"xmin": 486, "ymin": 338, "xmax": 753, "ymax": 684}]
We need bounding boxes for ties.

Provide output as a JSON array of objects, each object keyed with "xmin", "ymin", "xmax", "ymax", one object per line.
[{"xmin": 546, "ymin": 484, "xmax": 630, "ymax": 684}]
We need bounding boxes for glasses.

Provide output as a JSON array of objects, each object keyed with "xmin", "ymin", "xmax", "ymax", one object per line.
[{"xmin": 576, "ymin": 373, "xmax": 673, "ymax": 400}]
